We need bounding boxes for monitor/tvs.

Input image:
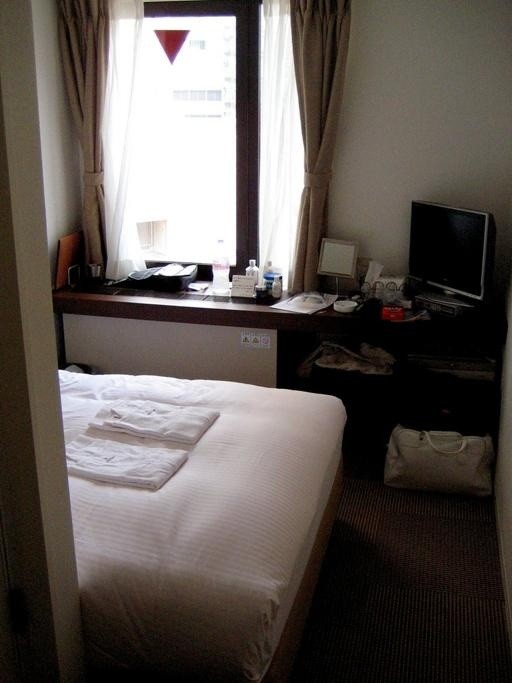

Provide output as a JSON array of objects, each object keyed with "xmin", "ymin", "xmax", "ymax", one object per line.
[{"xmin": 408, "ymin": 200, "xmax": 496, "ymax": 301}]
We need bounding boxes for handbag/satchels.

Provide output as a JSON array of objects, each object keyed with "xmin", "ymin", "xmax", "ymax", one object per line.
[
  {"xmin": 128, "ymin": 264, "xmax": 198, "ymax": 292},
  {"xmin": 384, "ymin": 425, "xmax": 493, "ymax": 495}
]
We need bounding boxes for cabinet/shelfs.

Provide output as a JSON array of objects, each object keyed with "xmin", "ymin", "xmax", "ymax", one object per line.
[{"xmin": 296, "ymin": 294, "xmax": 508, "ymax": 476}]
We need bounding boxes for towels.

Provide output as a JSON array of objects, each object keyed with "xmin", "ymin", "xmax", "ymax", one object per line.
[
  {"xmin": 65, "ymin": 434, "xmax": 188, "ymax": 491},
  {"xmin": 88, "ymin": 399, "xmax": 220, "ymax": 445}
]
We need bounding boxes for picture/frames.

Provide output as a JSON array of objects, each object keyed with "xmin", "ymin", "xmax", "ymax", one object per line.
[{"xmin": 317, "ymin": 237, "xmax": 360, "ymax": 280}]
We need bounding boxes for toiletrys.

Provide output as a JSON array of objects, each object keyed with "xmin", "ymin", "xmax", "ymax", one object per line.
[{"xmin": 245, "ymin": 259, "xmax": 283, "ymax": 299}]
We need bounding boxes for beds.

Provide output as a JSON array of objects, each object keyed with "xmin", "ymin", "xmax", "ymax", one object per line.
[{"xmin": 57, "ymin": 366, "xmax": 348, "ymax": 683}]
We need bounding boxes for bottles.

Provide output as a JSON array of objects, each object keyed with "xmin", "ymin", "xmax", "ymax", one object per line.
[{"xmin": 211, "ymin": 238, "xmax": 282, "ymax": 299}]
